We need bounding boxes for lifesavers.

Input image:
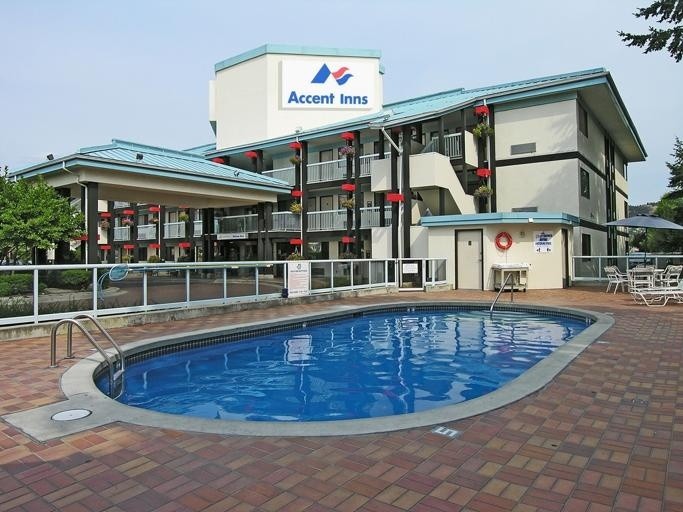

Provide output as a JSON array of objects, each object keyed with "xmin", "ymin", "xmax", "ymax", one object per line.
[{"xmin": 495, "ymin": 232, "xmax": 512, "ymax": 251}]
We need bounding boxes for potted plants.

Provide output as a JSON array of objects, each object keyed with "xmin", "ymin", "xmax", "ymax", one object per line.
[{"xmin": 474, "ymin": 185, "xmax": 493, "ymax": 198}]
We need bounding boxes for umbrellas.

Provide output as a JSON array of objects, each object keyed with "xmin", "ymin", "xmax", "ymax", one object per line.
[{"xmin": 599, "ymin": 213, "xmax": 682, "ymax": 269}]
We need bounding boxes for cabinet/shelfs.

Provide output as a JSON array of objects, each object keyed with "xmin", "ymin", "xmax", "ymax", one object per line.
[{"xmin": 492, "ymin": 264, "xmax": 528, "ymax": 292}]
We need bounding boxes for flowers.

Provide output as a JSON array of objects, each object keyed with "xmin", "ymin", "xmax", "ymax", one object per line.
[
  {"xmin": 289, "ymin": 202, "xmax": 303, "ymax": 213},
  {"xmin": 121, "ymin": 218, "xmax": 134, "ymax": 226},
  {"xmin": 339, "ymin": 146, "xmax": 353, "ymax": 155},
  {"xmin": 289, "ymin": 155, "xmax": 301, "ymax": 166}
]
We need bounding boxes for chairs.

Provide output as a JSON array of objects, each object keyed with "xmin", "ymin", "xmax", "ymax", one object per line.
[{"xmin": 603, "ymin": 264, "xmax": 682, "ymax": 307}]
